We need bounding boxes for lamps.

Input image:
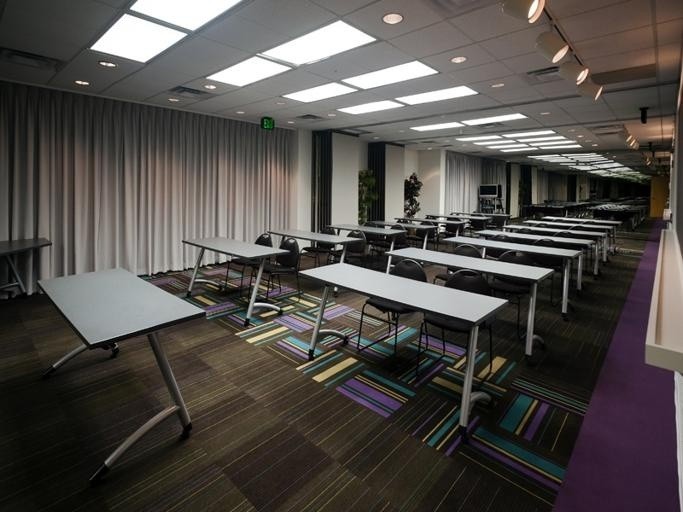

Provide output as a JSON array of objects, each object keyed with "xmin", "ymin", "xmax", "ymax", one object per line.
[
  {"xmin": 535, "ymin": 21, "xmax": 569, "ymax": 62},
  {"xmin": 500, "ymin": 0, "xmax": 545, "ymax": 24},
  {"xmin": 580, "ymin": 80, "xmax": 603, "ymax": 101},
  {"xmin": 643, "ymin": 156, "xmax": 660, "ymax": 175},
  {"xmin": 558, "ymin": 59, "xmax": 589, "ymax": 86},
  {"xmin": 621, "ymin": 131, "xmax": 640, "ymax": 149}
]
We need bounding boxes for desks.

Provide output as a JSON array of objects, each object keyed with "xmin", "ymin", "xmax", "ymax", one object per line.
[
  {"xmin": 0, "ymin": 237, "xmax": 53, "ymax": 297},
  {"xmin": 37, "ymin": 266, "xmax": 205, "ymax": 482}
]
